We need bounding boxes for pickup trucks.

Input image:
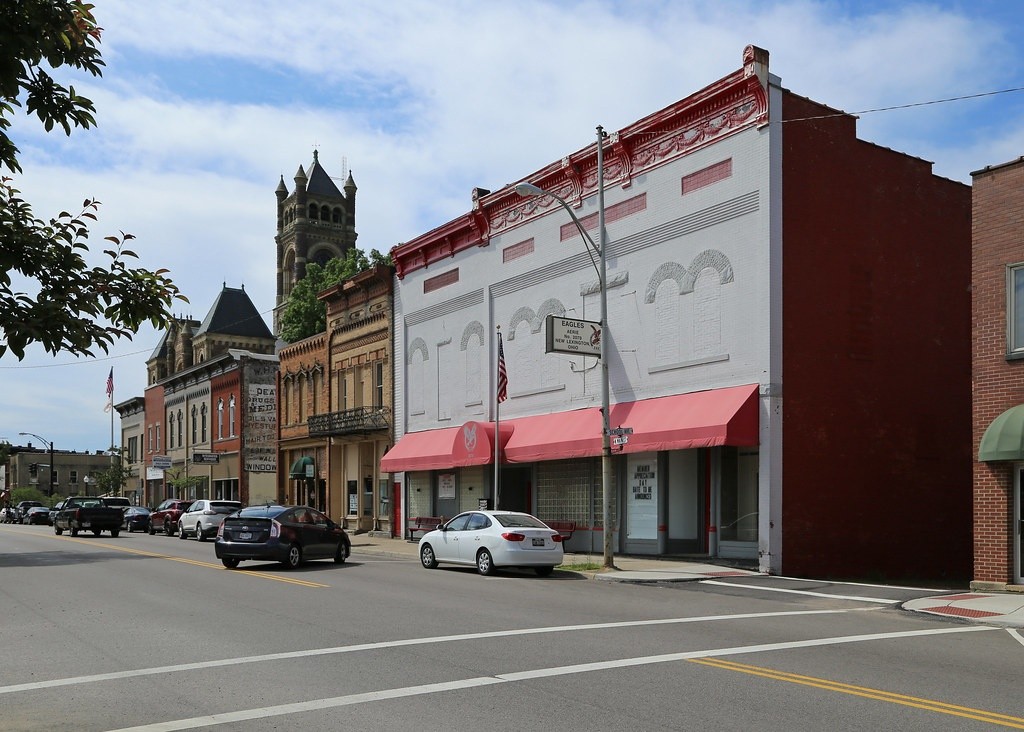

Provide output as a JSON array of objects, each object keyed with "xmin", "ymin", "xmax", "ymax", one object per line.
[{"xmin": 49, "ymin": 494, "xmax": 125, "ymax": 538}]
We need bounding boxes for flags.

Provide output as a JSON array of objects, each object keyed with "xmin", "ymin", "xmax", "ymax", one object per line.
[
  {"xmin": 497, "ymin": 332, "xmax": 508, "ymax": 404},
  {"xmin": 107, "ymin": 366, "xmax": 114, "ymax": 398}
]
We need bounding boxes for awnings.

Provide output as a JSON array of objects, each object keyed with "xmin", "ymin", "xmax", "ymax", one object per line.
[
  {"xmin": 288, "ymin": 456, "xmax": 315, "ymax": 480},
  {"xmin": 978, "ymin": 402, "xmax": 1024, "ymax": 463},
  {"xmin": 381, "ymin": 383, "xmax": 760, "ymax": 474}
]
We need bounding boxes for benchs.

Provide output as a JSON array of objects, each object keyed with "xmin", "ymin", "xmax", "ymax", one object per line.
[
  {"xmin": 543, "ymin": 521, "xmax": 576, "ymax": 552},
  {"xmin": 407, "ymin": 515, "xmax": 448, "ymax": 544}
]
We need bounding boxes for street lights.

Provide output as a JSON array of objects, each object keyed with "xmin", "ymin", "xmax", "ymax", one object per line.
[
  {"xmin": 512, "ymin": 124, "xmax": 615, "ymax": 566},
  {"xmin": 84, "ymin": 475, "xmax": 90, "ymax": 496},
  {"xmin": 18, "ymin": 432, "xmax": 54, "ymax": 498}
]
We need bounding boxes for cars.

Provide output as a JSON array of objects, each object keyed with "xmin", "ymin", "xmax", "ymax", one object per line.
[
  {"xmin": 0, "ymin": 498, "xmax": 96, "ymax": 527},
  {"xmin": 417, "ymin": 509, "xmax": 564, "ymax": 578},
  {"xmin": 119, "ymin": 506, "xmax": 151, "ymax": 534},
  {"xmin": 213, "ymin": 504, "xmax": 352, "ymax": 571}
]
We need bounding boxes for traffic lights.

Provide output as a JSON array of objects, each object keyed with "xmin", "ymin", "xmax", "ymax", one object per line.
[
  {"xmin": 28, "ymin": 463, "xmax": 33, "ymax": 473},
  {"xmin": 32, "ymin": 463, "xmax": 37, "ymax": 472}
]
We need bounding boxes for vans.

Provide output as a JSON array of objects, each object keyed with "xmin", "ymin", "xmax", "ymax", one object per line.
[{"xmin": 97, "ymin": 496, "xmax": 131, "ymax": 511}]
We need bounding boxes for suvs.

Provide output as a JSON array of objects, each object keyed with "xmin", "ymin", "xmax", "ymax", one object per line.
[
  {"xmin": 145, "ymin": 497, "xmax": 202, "ymax": 537},
  {"xmin": 176, "ymin": 497, "xmax": 247, "ymax": 542}
]
